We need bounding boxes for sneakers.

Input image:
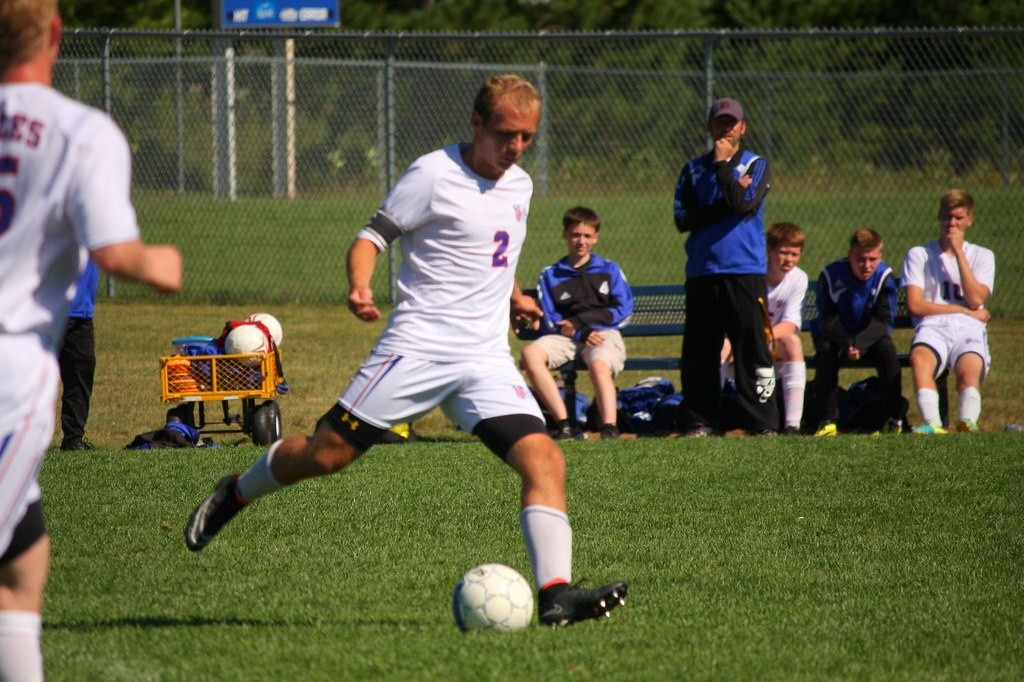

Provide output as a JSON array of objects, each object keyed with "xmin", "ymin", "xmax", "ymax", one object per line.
[
  {"xmin": 551, "ymin": 419, "xmax": 575, "ymax": 441},
  {"xmin": 539, "ymin": 581, "xmax": 628, "ymax": 626},
  {"xmin": 601, "ymin": 425, "xmax": 619, "ymax": 439},
  {"xmin": 184, "ymin": 474, "xmax": 246, "ymax": 552}
]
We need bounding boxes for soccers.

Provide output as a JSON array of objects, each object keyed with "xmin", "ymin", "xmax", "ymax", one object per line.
[
  {"xmin": 225, "ymin": 325, "xmax": 268, "ymax": 364},
  {"xmin": 454, "ymin": 563, "xmax": 534, "ymax": 631},
  {"xmin": 245, "ymin": 313, "xmax": 282, "ymax": 350}
]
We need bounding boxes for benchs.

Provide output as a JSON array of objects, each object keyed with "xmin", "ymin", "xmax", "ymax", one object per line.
[{"xmin": 511, "ymin": 276, "xmax": 948, "ymax": 420}]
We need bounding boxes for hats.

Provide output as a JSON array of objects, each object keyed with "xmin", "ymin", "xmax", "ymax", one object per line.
[{"xmin": 709, "ymin": 98, "xmax": 744, "ymax": 122}]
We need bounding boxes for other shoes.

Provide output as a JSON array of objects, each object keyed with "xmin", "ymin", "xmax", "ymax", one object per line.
[
  {"xmin": 784, "ymin": 426, "xmax": 801, "ymax": 437},
  {"xmin": 958, "ymin": 418, "xmax": 978, "ymax": 432},
  {"xmin": 760, "ymin": 429, "xmax": 777, "ymax": 436},
  {"xmin": 813, "ymin": 422, "xmax": 837, "ymax": 437},
  {"xmin": 871, "ymin": 418, "xmax": 904, "ymax": 436},
  {"xmin": 675, "ymin": 426, "xmax": 708, "ymax": 438},
  {"xmin": 911, "ymin": 423, "xmax": 948, "ymax": 434}
]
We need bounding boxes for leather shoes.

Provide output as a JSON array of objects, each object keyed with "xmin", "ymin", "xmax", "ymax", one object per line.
[{"xmin": 60, "ymin": 440, "xmax": 94, "ymax": 451}]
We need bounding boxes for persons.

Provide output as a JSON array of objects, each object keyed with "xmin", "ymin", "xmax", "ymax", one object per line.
[
  {"xmin": 522, "ymin": 206, "xmax": 634, "ymax": 443},
  {"xmin": 719, "ymin": 220, "xmax": 807, "ymax": 437},
  {"xmin": 187, "ymin": 71, "xmax": 629, "ymax": 625},
  {"xmin": 816, "ymin": 227, "xmax": 912, "ymax": 433},
  {"xmin": 672, "ymin": 101, "xmax": 784, "ymax": 440},
  {"xmin": 0, "ymin": 0, "xmax": 181, "ymax": 682},
  {"xmin": 902, "ymin": 188, "xmax": 994, "ymax": 433}
]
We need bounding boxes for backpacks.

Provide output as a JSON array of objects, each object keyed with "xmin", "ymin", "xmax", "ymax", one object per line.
[{"xmin": 587, "ymin": 376, "xmax": 910, "ymax": 434}]
